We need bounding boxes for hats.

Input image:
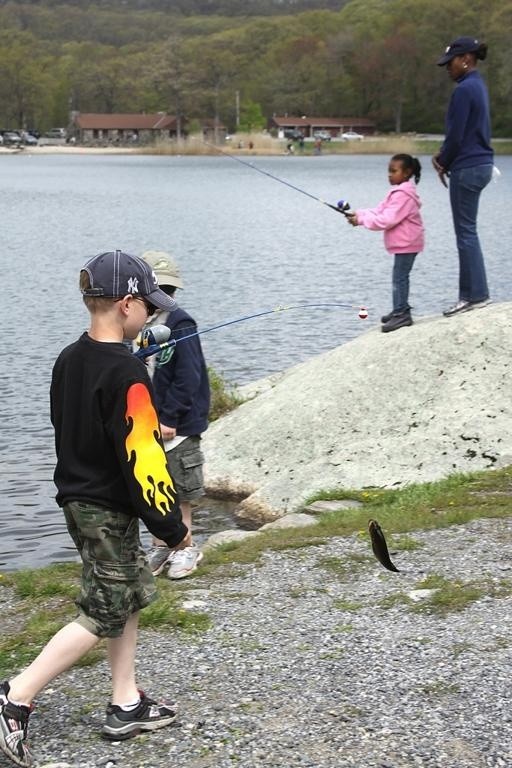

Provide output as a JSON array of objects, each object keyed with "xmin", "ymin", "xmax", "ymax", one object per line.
[
  {"xmin": 435, "ymin": 38, "xmax": 480, "ymax": 68},
  {"xmin": 79, "ymin": 248, "xmax": 180, "ymax": 313},
  {"xmin": 138, "ymin": 250, "xmax": 185, "ymax": 291}
]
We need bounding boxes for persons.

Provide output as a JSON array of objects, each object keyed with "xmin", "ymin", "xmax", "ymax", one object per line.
[
  {"xmin": 429, "ymin": 35, "xmax": 494, "ymax": 319},
  {"xmin": 1, "ymin": 249, "xmax": 180, "ymax": 768},
  {"xmin": 298, "ymin": 134, "xmax": 304, "ymax": 153},
  {"xmin": 344, "ymin": 153, "xmax": 427, "ymax": 335},
  {"xmin": 286, "ymin": 138, "xmax": 295, "ymax": 155},
  {"xmin": 135, "ymin": 248, "xmax": 212, "ymax": 583},
  {"xmin": 312, "ymin": 136, "xmax": 323, "ymax": 154}
]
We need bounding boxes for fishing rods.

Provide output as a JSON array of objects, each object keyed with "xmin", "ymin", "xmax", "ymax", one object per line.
[
  {"xmin": 134, "ymin": 303, "xmax": 368, "ymax": 365},
  {"xmin": 193, "ymin": 138, "xmax": 354, "ymax": 217}
]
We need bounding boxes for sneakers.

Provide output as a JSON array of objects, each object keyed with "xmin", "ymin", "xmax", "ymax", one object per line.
[
  {"xmin": 442, "ymin": 297, "xmax": 492, "ymax": 318},
  {"xmin": 380, "ymin": 309, "xmax": 413, "ymax": 333},
  {"xmin": 0, "ymin": 681, "xmax": 36, "ymax": 767},
  {"xmin": 99, "ymin": 691, "xmax": 180, "ymax": 741},
  {"xmin": 167, "ymin": 542, "xmax": 204, "ymax": 580},
  {"xmin": 147, "ymin": 544, "xmax": 174, "ymax": 577}
]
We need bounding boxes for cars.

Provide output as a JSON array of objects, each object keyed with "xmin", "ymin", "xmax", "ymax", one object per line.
[
  {"xmin": 284, "ymin": 128, "xmax": 364, "ymax": 142},
  {"xmin": 0, "ymin": 126, "xmax": 68, "ymax": 146}
]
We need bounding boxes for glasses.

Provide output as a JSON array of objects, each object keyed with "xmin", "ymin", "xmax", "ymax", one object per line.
[{"xmin": 114, "ymin": 297, "xmax": 155, "ymax": 316}]
